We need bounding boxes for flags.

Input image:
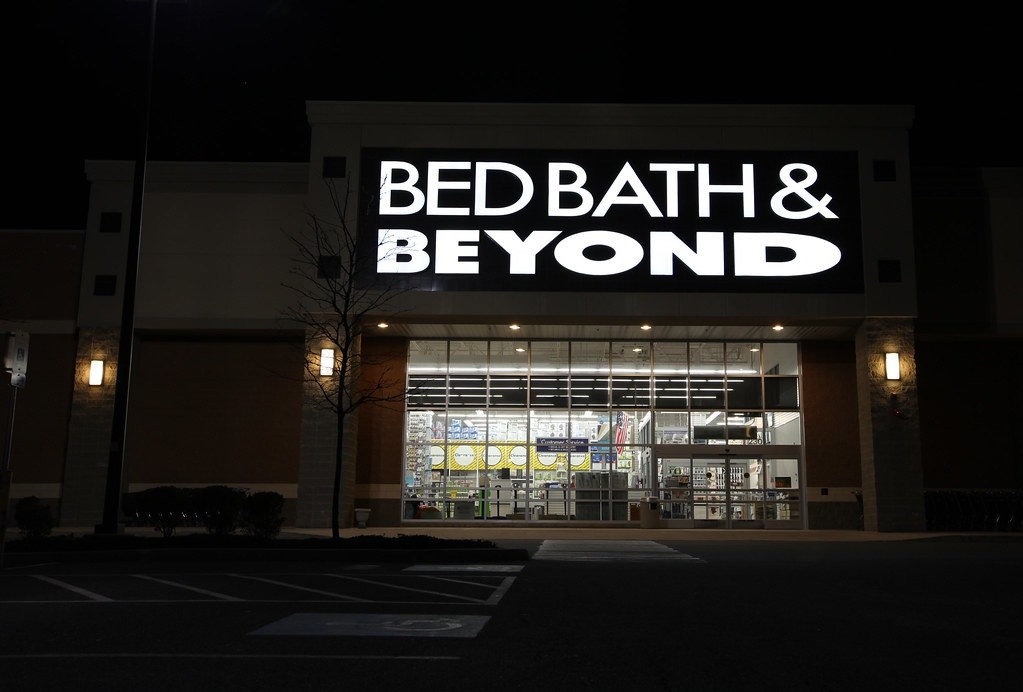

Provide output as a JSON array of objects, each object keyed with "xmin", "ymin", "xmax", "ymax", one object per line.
[{"xmin": 615, "ymin": 410, "xmax": 629, "ymax": 456}]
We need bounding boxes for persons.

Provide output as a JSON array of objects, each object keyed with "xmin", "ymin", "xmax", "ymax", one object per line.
[
  {"xmin": 479, "ymin": 470, "xmax": 491, "ymax": 487},
  {"xmin": 570, "ymin": 475, "xmax": 575, "ymax": 487}
]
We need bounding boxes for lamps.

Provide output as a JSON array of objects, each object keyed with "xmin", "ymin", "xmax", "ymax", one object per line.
[
  {"xmin": 320, "ymin": 348, "xmax": 334, "ymax": 375},
  {"xmin": 89, "ymin": 361, "xmax": 103, "ymax": 386},
  {"xmin": 885, "ymin": 352, "xmax": 900, "ymax": 381}
]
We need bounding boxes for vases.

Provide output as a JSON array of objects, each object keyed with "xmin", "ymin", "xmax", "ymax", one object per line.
[{"xmin": 354, "ymin": 508, "xmax": 371, "ymax": 528}]
